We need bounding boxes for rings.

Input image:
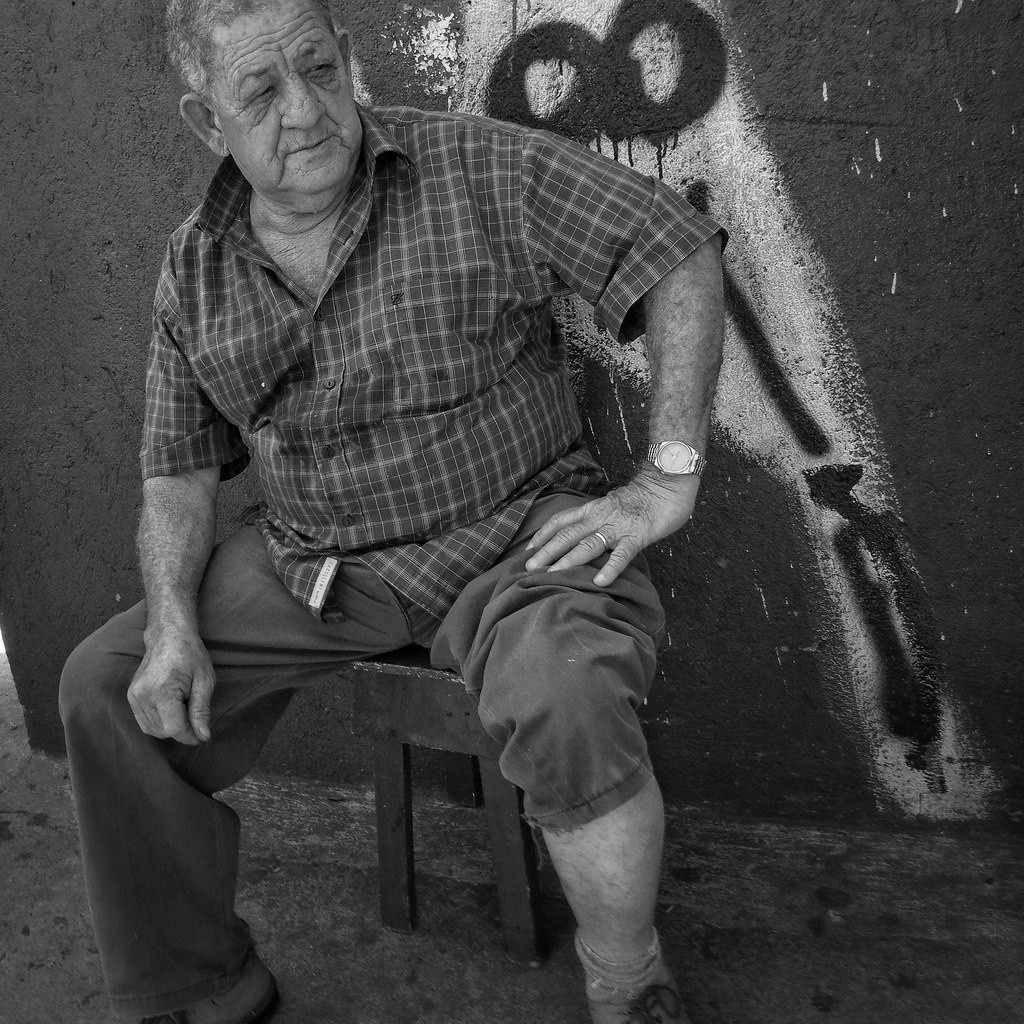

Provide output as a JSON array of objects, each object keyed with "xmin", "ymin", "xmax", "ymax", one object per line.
[{"xmin": 594, "ymin": 532, "xmax": 609, "ymax": 550}]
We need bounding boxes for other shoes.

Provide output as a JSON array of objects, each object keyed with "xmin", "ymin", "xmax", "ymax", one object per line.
[
  {"xmin": 135, "ymin": 956, "xmax": 277, "ymax": 1024},
  {"xmin": 574, "ymin": 923, "xmax": 692, "ymax": 1024}
]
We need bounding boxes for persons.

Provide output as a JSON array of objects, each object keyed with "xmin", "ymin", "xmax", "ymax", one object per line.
[{"xmin": 56, "ymin": 0, "xmax": 729, "ymax": 1022}]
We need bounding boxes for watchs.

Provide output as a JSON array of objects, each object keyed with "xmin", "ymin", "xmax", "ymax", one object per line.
[{"xmin": 642, "ymin": 440, "xmax": 707, "ymax": 478}]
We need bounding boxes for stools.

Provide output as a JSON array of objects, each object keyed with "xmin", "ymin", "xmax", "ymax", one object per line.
[{"xmin": 353, "ymin": 645, "xmax": 558, "ymax": 965}]
